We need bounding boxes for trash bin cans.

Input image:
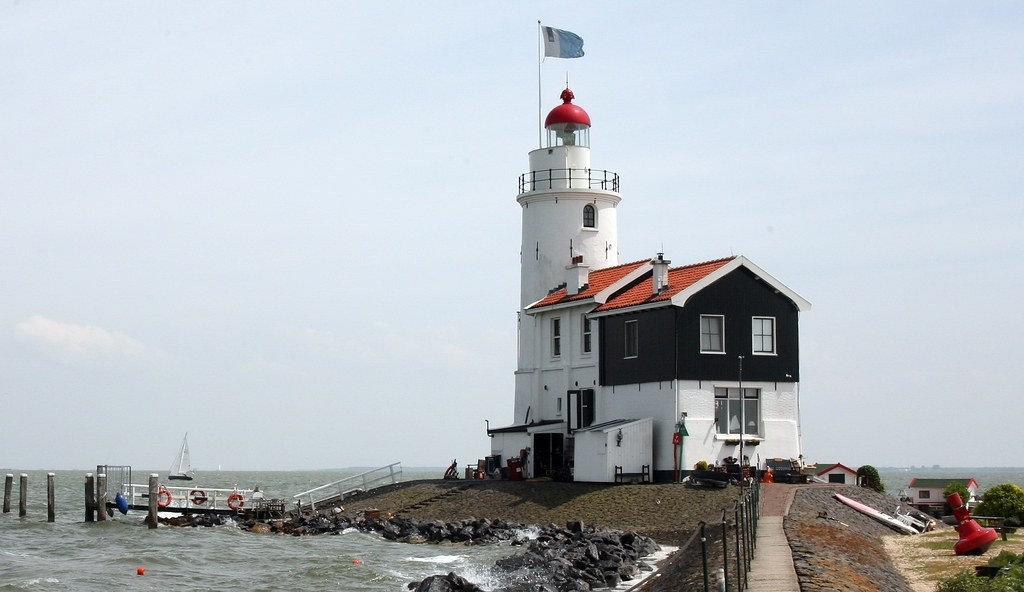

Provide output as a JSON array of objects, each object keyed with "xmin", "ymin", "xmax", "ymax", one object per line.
[{"xmin": 507, "ymin": 457, "xmax": 523, "ymax": 480}]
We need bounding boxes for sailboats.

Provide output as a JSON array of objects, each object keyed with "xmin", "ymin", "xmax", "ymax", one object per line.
[{"xmin": 168, "ymin": 431, "xmax": 194, "ymax": 480}]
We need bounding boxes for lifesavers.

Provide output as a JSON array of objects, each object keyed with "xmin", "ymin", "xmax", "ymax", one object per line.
[
  {"xmin": 189, "ymin": 490, "xmax": 207, "ymax": 506},
  {"xmin": 155, "ymin": 489, "xmax": 174, "ymax": 508},
  {"xmin": 227, "ymin": 492, "xmax": 245, "ymax": 513}
]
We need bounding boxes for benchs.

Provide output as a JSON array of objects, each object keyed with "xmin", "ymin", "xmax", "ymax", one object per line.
[
  {"xmin": 614, "ymin": 464, "xmax": 651, "ymax": 484},
  {"xmin": 954, "ymin": 517, "xmax": 1007, "ymax": 541}
]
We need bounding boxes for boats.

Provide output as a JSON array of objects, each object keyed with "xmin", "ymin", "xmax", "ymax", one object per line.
[{"xmin": 691, "ymin": 469, "xmax": 729, "ymax": 488}]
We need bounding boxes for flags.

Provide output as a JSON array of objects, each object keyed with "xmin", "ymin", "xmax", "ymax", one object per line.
[{"xmin": 541, "ymin": 26, "xmax": 585, "ymax": 58}]
